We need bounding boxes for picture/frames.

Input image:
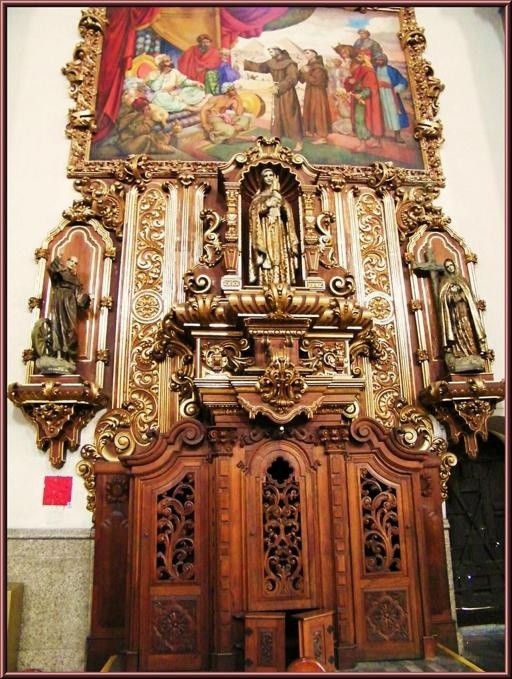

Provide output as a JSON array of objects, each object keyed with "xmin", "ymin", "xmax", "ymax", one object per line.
[{"xmin": 61, "ymin": 7, "xmax": 448, "ymax": 190}]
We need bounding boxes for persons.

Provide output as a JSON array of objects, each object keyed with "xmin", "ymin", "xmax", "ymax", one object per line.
[
  {"xmin": 296, "ymin": 48, "xmax": 331, "ymax": 145},
  {"xmin": 326, "ymin": 27, "xmax": 409, "ymax": 153},
  {"xmin": 437, "ymin": 258, "xmax": 487, "ymax": 358},
  {"xmin": 236, "ymin": 44, "xmax": 305, "ymax": 153},
  {"xmin": 247, "ymin": 166, "xmax": 301, "ymax": 286},
  {"xmin": 177, "ymin": 32, "xmax": 226, "ymax": 83},
  {"xmin": 46, "ymin": 246, "xmax": 88, "ymax": 364},
  {"xmin": 144, "ymin": 52, "xmax": 213, "ymax": 114}
]
